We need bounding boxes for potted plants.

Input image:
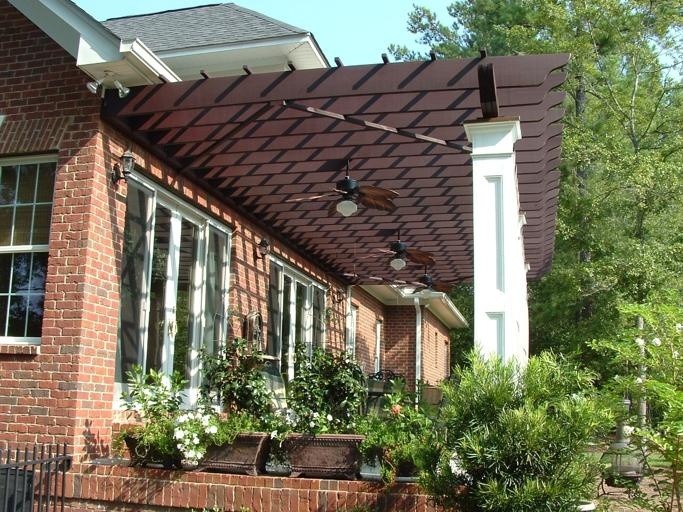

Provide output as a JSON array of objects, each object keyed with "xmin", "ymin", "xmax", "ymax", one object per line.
[{"xmin": 111, "ymin": 361, "xmax": 191, "ymax": 470}]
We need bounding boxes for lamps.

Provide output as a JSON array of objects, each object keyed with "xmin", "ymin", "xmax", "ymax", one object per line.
[
  {"xmin": 253, "ymin": 239, "xmax": 269, "ymax": 266},
  {"xmin": 112, "ymin": 142, "xmax": 137, "ymax": 185},
  {"xmin": 111, "ymin": 74, "xmax": 131, "ymax": 99},
  {"xmin": 335, "ymin": 200, "xmax": 358, "ymax": 217},
  {"xmin": 389, "ymin": 258, "xmax": 406, "ymax": 272},
  {"xmin": 85, "ymin": 72, "xmax": 109, "ymax": 95},
  {"xmin": 332, "ymin": 288, "xmax": 345, "ymax": 304},
  {"xmin": 420, "ymin": 288, "xmax": 433, "ymax": 300}
]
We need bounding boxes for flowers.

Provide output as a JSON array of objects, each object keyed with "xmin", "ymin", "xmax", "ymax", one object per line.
[
  {"xmin": 359, "ymin": 396, "xmax": 444, "ymax": 465},
  {"xmin": 270, "ymin": 403, "xmax": 367, "ymax": 470},
  {"xmin": 172, "ymin": 404, "xmax": 262, "ymax": 468}
]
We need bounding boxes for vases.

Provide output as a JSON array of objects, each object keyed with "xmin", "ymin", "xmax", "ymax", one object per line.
[
  {"xmin": 197, "ymin": 431, "xmax": 272, "ymax": 476},
  {"xmin": 376, "ymin": 447, "xmax": 422, "ymax": 483},
  {"xmin": 273, "ymin": 432, "xmax": 367, "ymax": 481}
]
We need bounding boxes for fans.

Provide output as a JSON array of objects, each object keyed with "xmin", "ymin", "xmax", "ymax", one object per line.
[
  {"xmin": 372, "ymin": 227, "xmax": 436, "ymax": 272},
  {"xmin": 403, "ymin": 265, "xmax": 453, "ymax": 295},
  {"xmin": 279, "ymin": 156, "xmax": 400, "ymax": 217}
]
